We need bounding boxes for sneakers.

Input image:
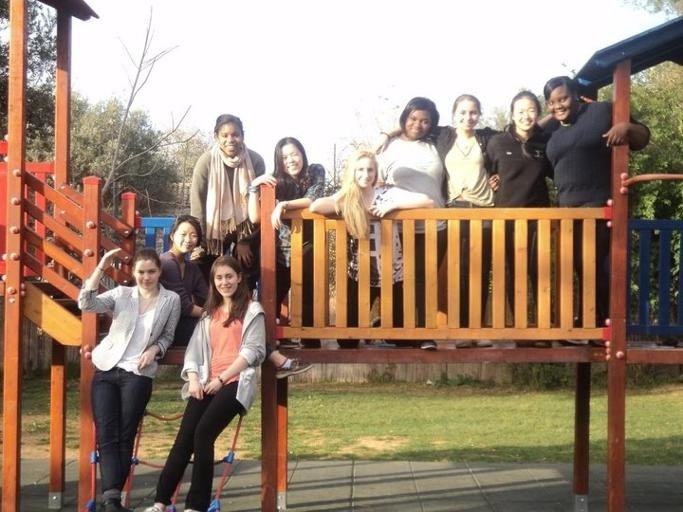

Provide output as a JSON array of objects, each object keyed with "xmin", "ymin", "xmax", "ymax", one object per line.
[
  {"xmin": 419, "ymin": 341, "xmax": 436, "ymax": 349},
  {"xmin": 97, "ymin": 497, "xmax": 165, "ymax": 512},
  {"xmin": 363, "ymin": 317, "xmax": 381, "ymax": 344},
  {"xmin": 515, "ymin": 339, "xmax": 551, "ymax": 348},
  {"xmin": 455, "ymin": 339, "xmax": 492, "ymax": 348},
  {"xmin": 276, "ymin": 359, "xmax": 312, "ymax": 379},
  {"xmin": 561, "ymin": 339, "xmax": 606, "ymax": 346}
]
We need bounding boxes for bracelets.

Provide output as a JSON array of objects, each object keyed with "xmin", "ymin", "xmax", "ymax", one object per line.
[
  {"xmin": 216, "ymin": 375, "xmax": 227, "ymax": 388},
  {"xmin": 284, "ymin": 201, "xmax": 288, "ymax": 209},
  {"xmin": 248, "ymin": 186, "xmax": 260, "ymax": 192},
  {"xmin": 380, "ymin": 130, "xmax": 391, "ymax": 142},
  {"xmin": 95, "ymin": 265, "xmax": 108, "ymax": 273}
]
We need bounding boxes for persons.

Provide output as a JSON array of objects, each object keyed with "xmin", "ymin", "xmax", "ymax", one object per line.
[
  {"xmin": 247, "ymin": 137, "xmax": 326, "ymax": 348},
  {"xmin": 158, "ymin": 213, "xmax": 314, "ymax": 380},
  {"xmin": 308, "ymin": 150, "xmax": 434, "ymax": 349},
  {"xmin": 189, "ymin": 113, "xmax": 299, "ymax": 348},
  {"xmin": 368, "ymin": 94, "xmax": 594, "ymax": 351},
  {"xmin": 485, "ymin": 91, "xmax": 555, "ymax": 345},
  {"xmin": 488, "ymin": 74, "xmax": 650, "ymax": 345},
  {"xmin": 140, "ymin": 254, "xmax": 267, "ymax": 512},
  {"xmin": 76, "ymin": 246, "xmax": 182, "ymax": 511},
  {"xmin": 372, "ymin": 97, "xmax": 446, "ymax": 349}
]
